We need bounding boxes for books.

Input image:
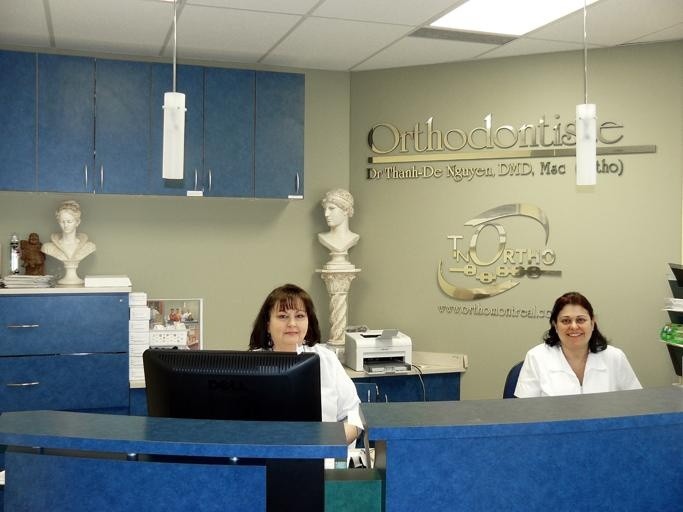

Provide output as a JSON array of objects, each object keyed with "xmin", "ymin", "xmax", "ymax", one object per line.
[{"xmin": 656, "ymin": 297, "xmax": 683, "ymax": 345}]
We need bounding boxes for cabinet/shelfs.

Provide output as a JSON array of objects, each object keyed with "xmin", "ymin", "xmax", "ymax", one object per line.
[
  {"xmin": 150, "ymin": 62, "xmax": 254, "ymax": 198},
  {"xmin": 0, "ymin": 49, "xmax": 36, "ymax": 192},
  {"xmin": 252, "ymin": 70, "xmax": 306, "ymax": 199},
  {"xmin": 0, "ymin": 293, "xmax": 129, "ymax": 413},
  {"xmin": 37, "ymin": 52, "xmax": 149, "ymax": 194}
]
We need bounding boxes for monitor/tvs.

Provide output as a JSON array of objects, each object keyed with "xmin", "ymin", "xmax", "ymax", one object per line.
[{"xmin": 142, "ymin": 349, "xmax": 322, "ymax": 423}]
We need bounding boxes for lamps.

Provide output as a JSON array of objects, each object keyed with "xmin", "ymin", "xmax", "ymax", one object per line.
[
  {"xmin": 574, "ymin": 1, "xmax": 599, "ymax": 188},
  {"xmin": 158, "ymin": 0, "xmax": 186, "ymax": 181}
]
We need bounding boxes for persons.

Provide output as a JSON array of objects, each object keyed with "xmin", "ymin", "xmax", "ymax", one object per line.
[
  {"xmin": 249, "ymin": 284, "xmax": 365, "ymax": 447},
  {"xmin": 513, "ymin": 292, "xmax": 643, "ymax": 398},
  {"xmin": 40, "ymin": 200, "xmax": 96, "ymax": 262},
  {"xmin": 148, "ymin": 305, "xmax": 193, "ymax": 325},
  {"xmin": 317, "ymin": 188, "xmax": 360, "ymax": 251}
]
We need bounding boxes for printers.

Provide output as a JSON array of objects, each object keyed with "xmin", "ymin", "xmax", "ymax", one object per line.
[{"xmin": 344, "ymin": 328, "xmax": 413, "ymax": 375}]
